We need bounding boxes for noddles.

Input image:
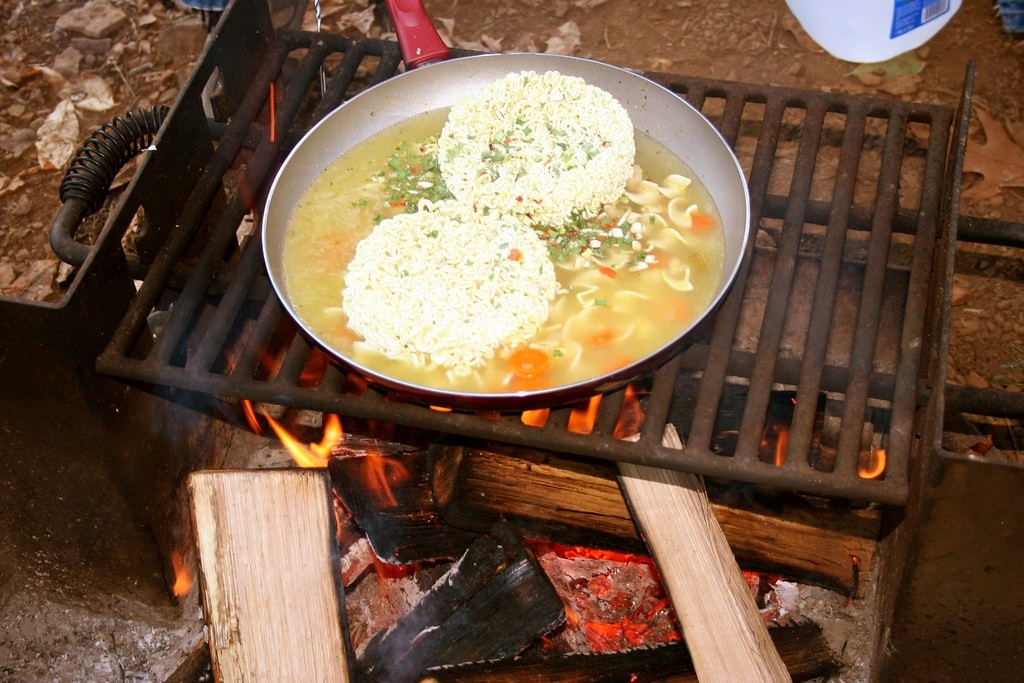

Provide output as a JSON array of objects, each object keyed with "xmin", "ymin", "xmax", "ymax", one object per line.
[{"xmin": 341, "ymin": 197, "xmax": 558, "ymax": 373}]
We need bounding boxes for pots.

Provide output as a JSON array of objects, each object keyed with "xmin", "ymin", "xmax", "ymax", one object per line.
[{"xmin": 261, "ymin": 2, "xmax": 752, "ymax": 409}]
